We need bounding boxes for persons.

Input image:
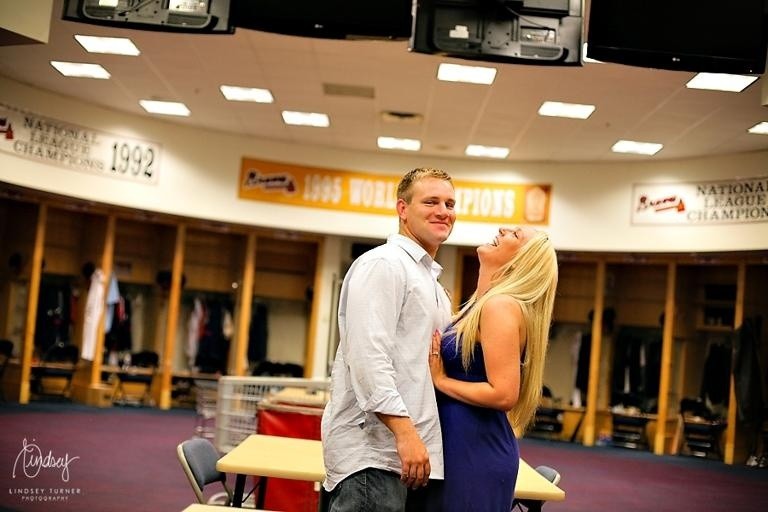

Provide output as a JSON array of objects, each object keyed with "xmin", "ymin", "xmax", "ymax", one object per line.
[
  {"xmin": 430, "ymin": 226, "xmax": 561, "ymax": 510},
  {"xmin": 320, "ymin": 167, "xmax": 459, "ymax": 511}
]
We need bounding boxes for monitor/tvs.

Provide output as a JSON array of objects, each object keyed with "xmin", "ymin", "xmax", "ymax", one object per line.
[
  {"xmin": 229, "ymin": 0, "xmax": 413, "ymax": 41},
  {"xmin": 587, "ymin": 0, "xmax": 768, "ymax": 74},
  {"xmin": 62, "ymin": 0, "xmax": 236, "ymax": 35},
  {"xmin": 406, "ymin": 0, "xmax": 585, "ymax": 66}
]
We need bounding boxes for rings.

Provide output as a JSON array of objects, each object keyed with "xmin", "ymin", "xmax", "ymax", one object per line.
[{"xmin": 432, "ymin": 350, "xmax": 438, "ymax": 356}]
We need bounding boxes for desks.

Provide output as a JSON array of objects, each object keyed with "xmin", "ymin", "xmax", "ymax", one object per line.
[
  {"xmin": 256, "ymin": 388, "xmax": 331, "ymax": 511},
  {"xmin": 216, "ymin": 434, "xmax": 565, "ymax": 511}
]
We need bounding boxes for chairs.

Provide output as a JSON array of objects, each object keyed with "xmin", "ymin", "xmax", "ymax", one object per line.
[
  {"xmin": 177, "ymin": 437, "xmax": 256, "ymax": 509},
  {"xmin": 526, "ymin": 408, "xmax": 728, "ymax": 462},
  {"xmin": 509, "ymin": 465, "xmax": 562, "ymax": 512}
]
